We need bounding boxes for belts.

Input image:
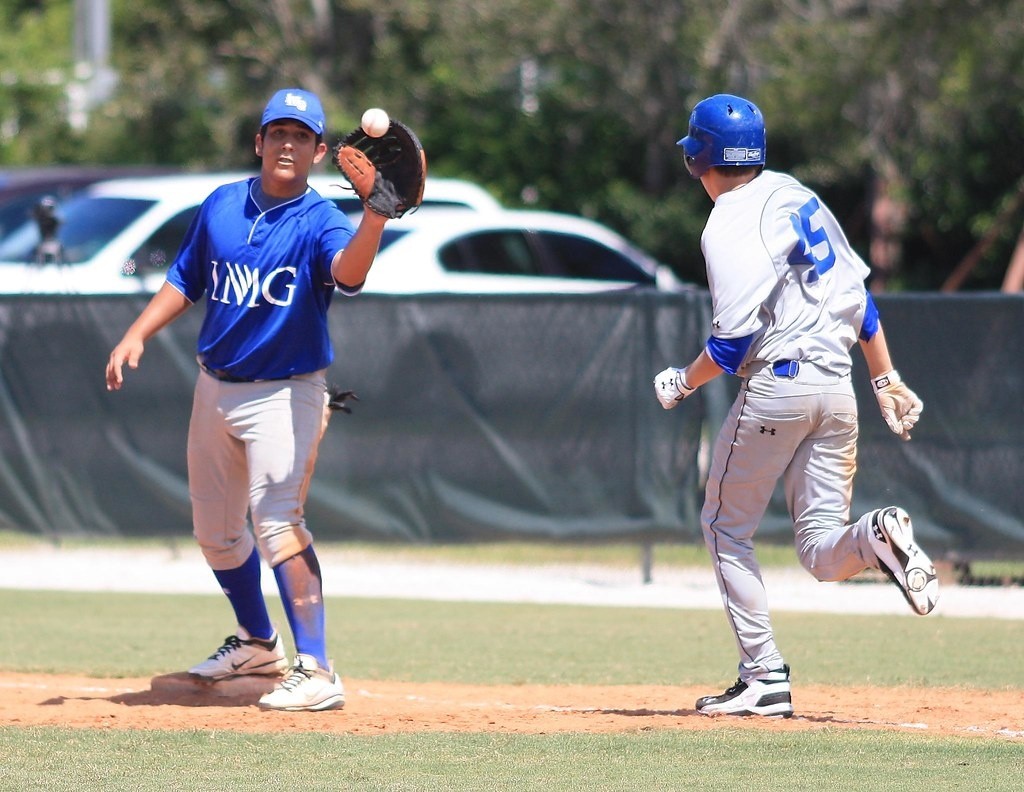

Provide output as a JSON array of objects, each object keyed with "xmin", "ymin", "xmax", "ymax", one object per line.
[
  {"xmin": 771, "ymin": 360, "xmax": 799, "ymax": 378},
  {"xmin": 202, "ymin": 362, "xmax": 291, "ymax": 383}
]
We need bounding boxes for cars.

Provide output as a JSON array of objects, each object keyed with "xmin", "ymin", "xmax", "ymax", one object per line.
[
  {"xmin": 0, "ymin": 171, "xmax": 504, "ymax": 294},
  {"xmin": 0, "ymin": 166, "xmax": 192, "ymax": 243},
  {"xmin": 329, "ymin": 207, "xmax": 690, "ymax": 296}
]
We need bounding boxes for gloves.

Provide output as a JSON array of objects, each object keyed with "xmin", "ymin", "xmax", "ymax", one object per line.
[
  {"xmin": 327, "ymin": 383, "xmax": 361, "ymax": 415},
  {"xmin": 871, "ymin": 370, "xmax": 923, "ymax": 441},
  {"xmin": 655, "ymin": 366, "xmax": 697, "ymax": 410}
]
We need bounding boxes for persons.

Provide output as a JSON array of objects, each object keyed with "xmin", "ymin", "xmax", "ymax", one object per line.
[
  {"xmin": 655, "ymin": 94, "xmax": 939, "ymax": 716},
  {"xmin": 104, "ymin": 88, "xmax": 387, "ymax": 713}
]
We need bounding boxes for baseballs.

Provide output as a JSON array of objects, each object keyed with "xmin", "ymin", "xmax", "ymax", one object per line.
[{"xmin": 359, "ymin": 107, "xmax": 391, "ymax": 139}]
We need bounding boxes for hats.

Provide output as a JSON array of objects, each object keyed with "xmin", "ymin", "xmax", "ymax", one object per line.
[{"xmin": 260, "ymin": 88, "xmax": 326, "ymax": 140}]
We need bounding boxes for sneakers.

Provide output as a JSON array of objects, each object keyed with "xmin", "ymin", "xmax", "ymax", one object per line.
[
  {"xmin": 259, "ymin": 654, "xmax": 345, "ymax": 711},
  {"xmin": 189, "ymin": 624, "xmax": 288, "ymax": 680},
  {"xmin": 858, "ymin": 504, "xmax": 940, "ymax": 616},
  {"xmin": 696, "ymin": 662, "xmax": 793, "ymax": 719}
]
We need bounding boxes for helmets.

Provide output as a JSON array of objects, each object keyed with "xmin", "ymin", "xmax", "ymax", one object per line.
[{"xmin": 675, "ymin": 94, "xmax": 766, "ymax": 177}]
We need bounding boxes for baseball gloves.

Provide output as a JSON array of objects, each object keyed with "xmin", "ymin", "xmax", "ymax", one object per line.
[{"xmin": 330, "ymin": 117, "xmax": 428, "ymax": 220}]
